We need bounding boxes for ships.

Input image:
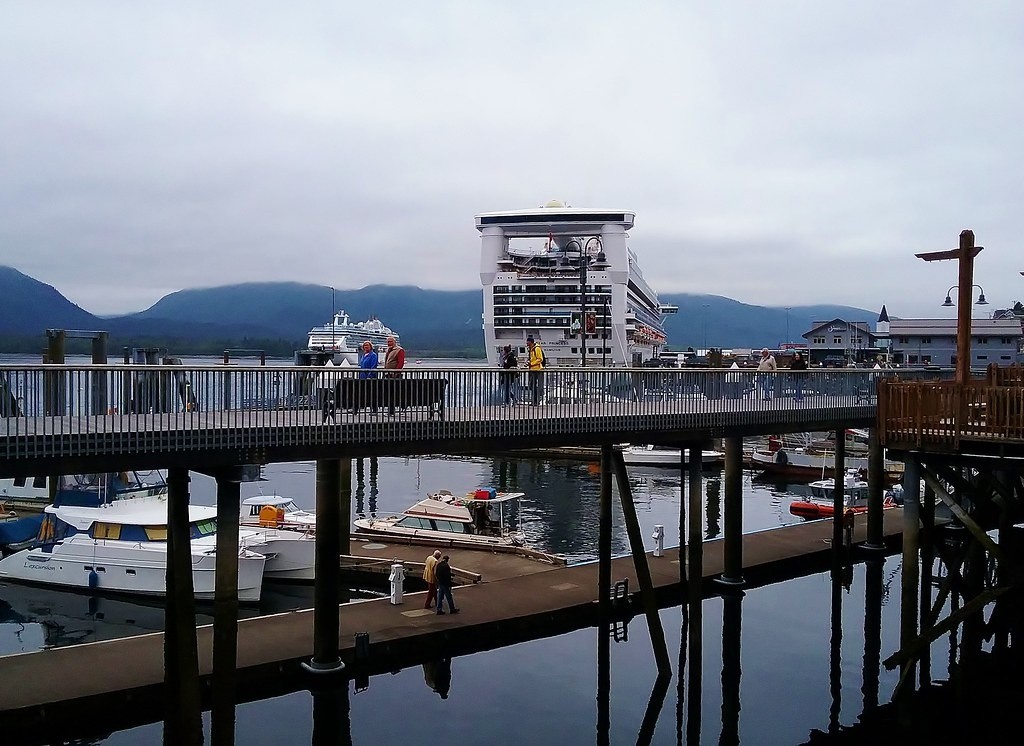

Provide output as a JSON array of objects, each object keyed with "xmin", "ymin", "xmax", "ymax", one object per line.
[{"xmin": 473, "ymin": 200, "xmax": 680, "ymax": 381}]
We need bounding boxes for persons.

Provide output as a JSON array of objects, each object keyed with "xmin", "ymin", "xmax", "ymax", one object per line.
[
  {"xmin": 858, "ymin": 357, "xmax": 930, "ymax": 388},
  {"xmin": 383, "ymin": 336, "xmax": 406, "ymax": 418},
  {"xmin": 434, "ymin": 556, "xmax": 461, "ymax": 615},
  {"xmin": 755, "ymin": 347, "xmax": 777, "ymax": 401},
  {"xmin": 786, "ymin": 351, "xmax": 809, "ymax": 403},
  {"xmin": 345, "ymin": 341, "xmax": 379, "ymax": 416},
  {"xmin": 498, "ymin": 343, "xmax": 519, "ymax": 408},
  {"xmin": 524, "ymin": 337, "xmax": 545, "ymax": 408},
  {"xmin": 422, "ymin": 550, "xmax": 443, "ymax": 608}
]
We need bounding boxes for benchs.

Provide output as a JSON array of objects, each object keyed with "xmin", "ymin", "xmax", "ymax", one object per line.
[
  {"xmin": 322, "ymin": 378, "xmax": 449, "ymax": 424},
  {"xmin": 855, "ymin": 384, "xmax": 877, "ymax": 405}
]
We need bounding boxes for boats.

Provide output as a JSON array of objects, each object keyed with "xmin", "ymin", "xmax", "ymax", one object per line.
[
  {"xmin": 351, "ymin": 487, "xmax": 527, "ymax": 550},
  {"xmin": 307, "ymin": 310, "xmax": 401, "ymax": 395},
  {"xmin": 515, "ymin": 424, "xmax": 938, "ymax": 527},
  {"xmin": 0, "ymin": 441, "xmax": 339, "ymax": 601}
]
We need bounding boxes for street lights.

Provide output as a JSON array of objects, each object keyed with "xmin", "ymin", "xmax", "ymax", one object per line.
[
  {"xmin": 551, "ymin": 235, "xmax": 611, "ymax": 406},
  {"xmin": 913, "ymin": 227, "xmax": 990, "ymax": 376}
]
